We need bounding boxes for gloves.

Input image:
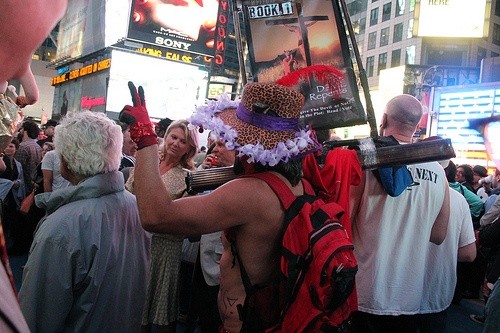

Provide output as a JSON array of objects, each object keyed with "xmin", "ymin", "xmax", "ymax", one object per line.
[{"xmin": 117, "ymin": 81, "xmax": 158, "ymax": 150}]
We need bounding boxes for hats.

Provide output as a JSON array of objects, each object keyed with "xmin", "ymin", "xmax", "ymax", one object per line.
[
  {"xmin": 43, "ymin": 120, "xmax": 57, "ymax": 127},
  {"xmin": 473, "ymin": 165, "xmax": 488, "ymax": 177},
  {"xmin": 191, "ymin": 81, "xmax": 321, "ymax": 167}
]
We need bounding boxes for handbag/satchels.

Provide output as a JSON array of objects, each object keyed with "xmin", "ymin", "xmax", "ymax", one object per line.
[{"xmin": 19, "ymin": 190, "xmax": 35, "ymax": 214}]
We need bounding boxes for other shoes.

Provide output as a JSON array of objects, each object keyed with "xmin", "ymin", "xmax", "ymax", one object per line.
[{"xmin": 471, "ymin": 314, "xmax": 484, "ymax": 325}]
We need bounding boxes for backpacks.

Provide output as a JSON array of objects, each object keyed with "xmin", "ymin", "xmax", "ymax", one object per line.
[{"xmin": 223, "ymin": 173, "xmax": 360, "ymax": 333}]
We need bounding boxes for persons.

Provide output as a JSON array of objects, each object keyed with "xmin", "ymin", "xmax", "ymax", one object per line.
[
  {"xmin": 0, "ymin": 0, "xmax": 500, "ymax": 333},
  {"xmin": 119, "ymin": 80, "xmax": 322, "ymax": 333},
  {"xmin": 17, "ymin": 107, "xmax": 154, "ymax": 333}
]
